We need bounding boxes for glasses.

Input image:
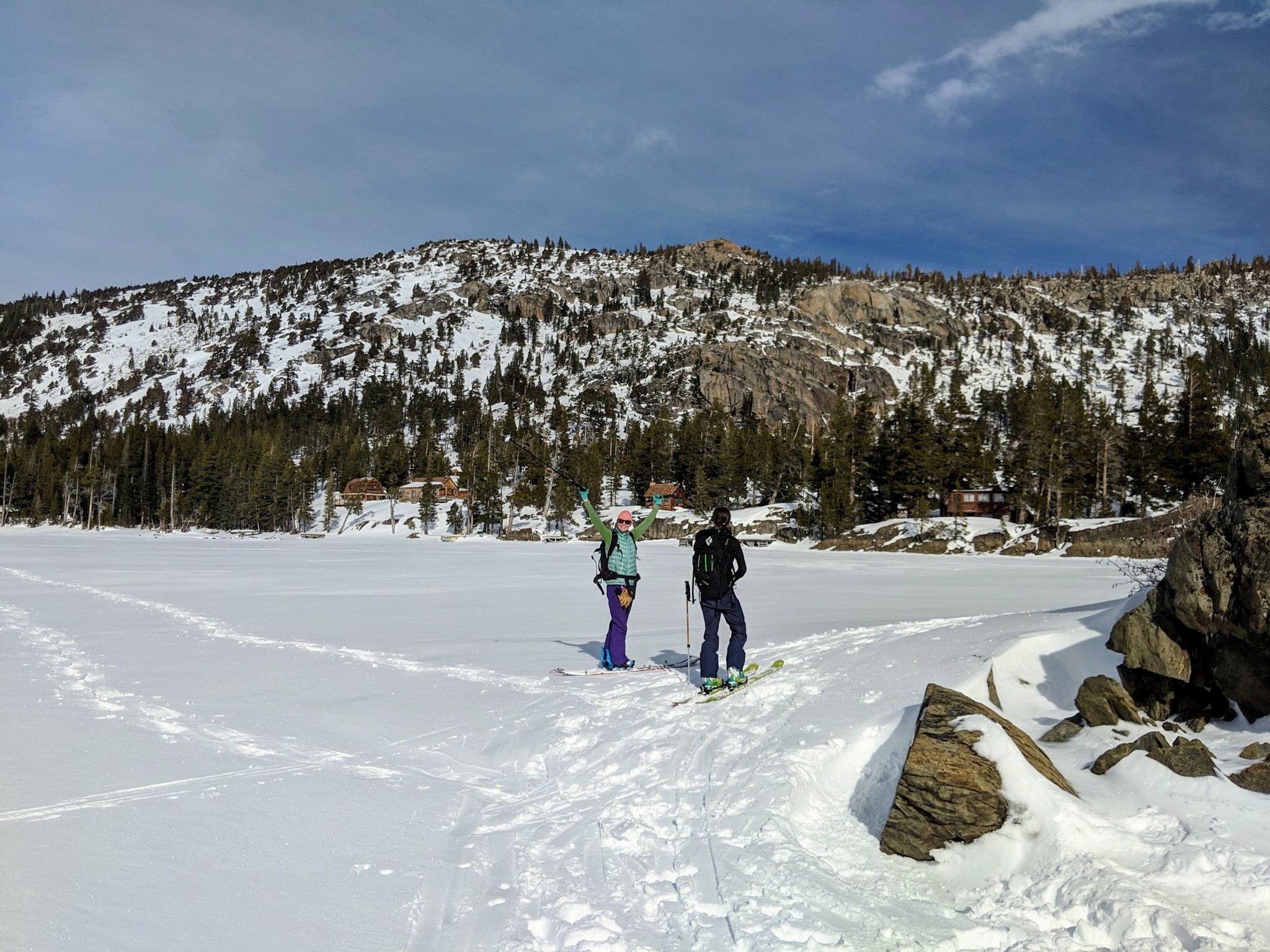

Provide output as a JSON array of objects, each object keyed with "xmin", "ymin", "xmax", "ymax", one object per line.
[{"xmin": 617, "ymin": 519, "xmax": 631, "ymax": 525}]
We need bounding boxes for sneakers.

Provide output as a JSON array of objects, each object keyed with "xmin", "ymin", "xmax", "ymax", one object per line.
[
  {"xmin": 728, "ymin": 666, "xmax": 746, "ymax": 685},
  {"xmin": 703, "ymin": 677, "xmax": 722, "ymax": 690}
]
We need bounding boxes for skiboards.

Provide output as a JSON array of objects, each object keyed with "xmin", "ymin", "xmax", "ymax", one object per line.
[
  {"xmin": 671, "ymin": 659, "xmax": 785, "ymax": 706},
  {"xmin": 555, "ymin": 656, "xmax": 702, "ymax": 676}
]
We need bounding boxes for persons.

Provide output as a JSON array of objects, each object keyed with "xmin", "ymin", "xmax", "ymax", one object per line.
[
  {"xmin": 579, "ymin": 485, "xmax": 664, "ymax": 671},
  {"xmin": 692, "ymin": 507, "xmax": 748, "ymax": 694}
]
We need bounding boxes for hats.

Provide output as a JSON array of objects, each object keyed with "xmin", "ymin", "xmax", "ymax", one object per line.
[{"xmin": 617, "ymin": 510, "xmax": 632, "ymax": 525}]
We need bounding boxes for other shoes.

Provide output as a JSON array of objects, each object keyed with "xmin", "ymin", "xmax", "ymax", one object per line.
[{"xmin": 613, "ymin": 660, "xmax": 635, "ymax": 668}]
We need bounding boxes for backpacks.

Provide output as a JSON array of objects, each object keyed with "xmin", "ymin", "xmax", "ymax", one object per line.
[
  {"xmin": 693, "ymin": 535, "xmax": 729, "ymax": 596},
  {"xmin": 598, "ymin": 531, "xmax": 640, "ymax": 581}
]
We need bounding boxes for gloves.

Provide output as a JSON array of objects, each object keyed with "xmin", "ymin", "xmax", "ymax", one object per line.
[
  {"xmin": 621, "ymin": 588, "xmax": 632, "ymax": 606},
  {"xmin": 653, "ymin": 494, "xmax": 664, "ymax": 506},
  {"xmin": 614, "ymin": 587, "xmax": 629, "ymax": 608},
  {"xmin": 578, "ymin": 486, "xmax": 589, "ymax": 501}
]
